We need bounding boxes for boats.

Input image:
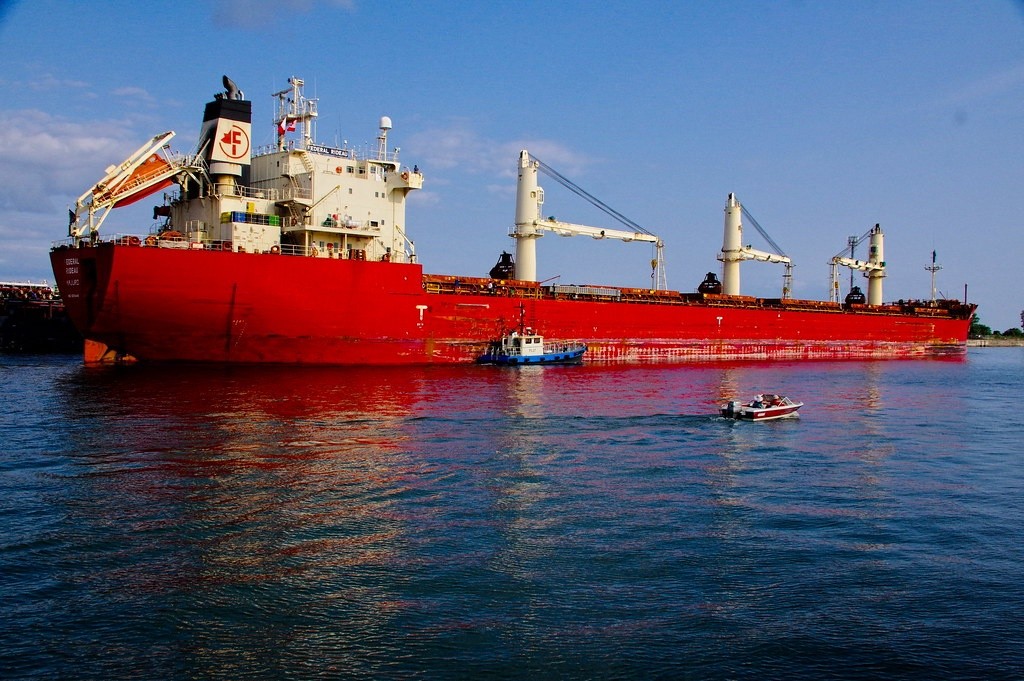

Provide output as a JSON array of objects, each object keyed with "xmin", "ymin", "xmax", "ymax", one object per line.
[
  {"xmin": 479, "ymin": 327, "xmax": 588, "ymax": 362},
  {"xmin": 49, "ymin": 72, "xmax": 980, "ymax": 367},
  {"xmin": 719, "ymin": 395, "xmax": 800, "ymax": 423}
]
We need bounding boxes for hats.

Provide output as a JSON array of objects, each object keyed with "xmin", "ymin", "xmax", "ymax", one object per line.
[
  {"xmin": 753, "ymin": 394, "xmax": 764, "ymax": 402},
  {"xmin": 772, "ymin": 395, "xmax": 779, "ymax": 399}
]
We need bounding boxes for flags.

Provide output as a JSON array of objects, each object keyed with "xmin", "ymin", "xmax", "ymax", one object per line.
[
  {"xmin": 278, "ymin": 118, "xmax": 286, "ymax": 136},
  {"xmin": 69, "ymin": 211, "xmax": 76, "ymax": 222},
  {"xmin": 287, "ymin": 119, "xmax": 297, "ymax": 132}
]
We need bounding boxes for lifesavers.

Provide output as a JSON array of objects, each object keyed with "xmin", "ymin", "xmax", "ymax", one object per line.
[
  {"xmin": 271, "ymin": 245, "xmax": 280, "ymax": 254},
  {"xmin": 337, "ymin": 166, "xmax": 342, "ymax": 173},
  {"xmin": 130, "ymin": 236, "xmax": 140, "ymax": 246},
  {"xmin": 327, "ymin": 243, "xmax": 334, "ymax": 250},
  {"xmin": 382, "ymin": 254, "xmax": 390, "ymax": 262},
  {"xmin": 145, "ymin": 237, "xmax": 155, "ymax": 246},
  {"xmin": 401, "ymin": 172, "xmax": 408, "ymax": 181},
  {"xmin": 223, "ymin": 242, "xmax": 232, "ymax": 251},
  {"xmin": 190, "ymin": 242, "xmax": 200, "ymax": 250},
  {"xmin": 292, "ymin": 219, "xmax": 297, "ymax": 224}
]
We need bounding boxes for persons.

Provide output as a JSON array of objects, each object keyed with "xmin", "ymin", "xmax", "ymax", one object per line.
[
  {"xmin": 753, "ymin": 395, "xmax": 764, "ymax": 408},
  {"xmin": 91, "ymin": 227, "xmax": 99, "ymax": 243},
  {"xmin": 414, "ymin": 165, "xmax": 418, "ymax": 173},
  {"xmin": 488, "ymin": 281, "xmax": 496, "ymax": 294},
  {"xmin": 770, "ymin": 395, "xmax": 785, "ymax": 406},
  {"xmin": 311, "ymin": 243, "xmax": 319, "ymax": 256}
]
